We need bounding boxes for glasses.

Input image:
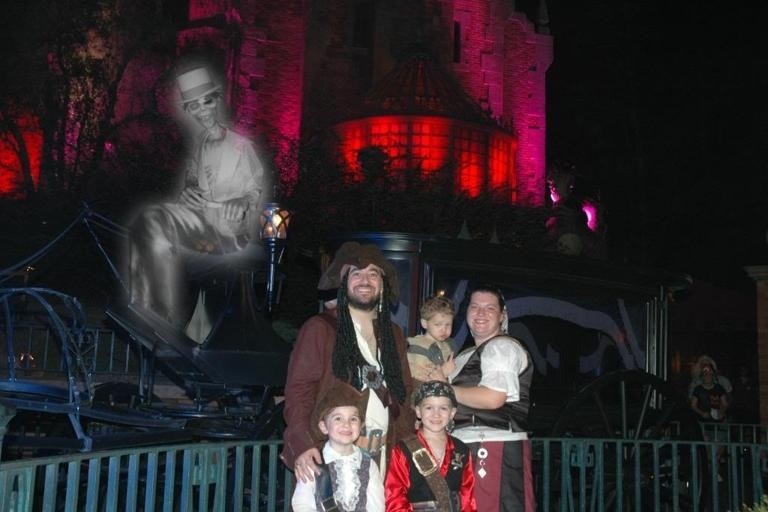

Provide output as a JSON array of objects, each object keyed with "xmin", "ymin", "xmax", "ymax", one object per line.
[{"xmin": 188, "ymin": 99, "xmax": 212, "ymax": 110}]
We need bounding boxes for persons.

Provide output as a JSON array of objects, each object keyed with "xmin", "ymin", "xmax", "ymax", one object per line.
[
  {"xmin": 383, "ymin": 380, "xmax": 480, "ymax": 511},
  {"xmin": 671, "ymin": 354, "xmax": 760, "ymax": 483},
  {"xmin": 125, "ymin": 62, "xmax": 269, "ymax": 362},
  {"xmin": 283, "ymin": 234, "xmax": 416, "ymax": 510},
  {"xmin": 290, "ymin": 386, "xmax": 387, "ymax": 512},
  {"xmin": 404, "ymin": 293, "xmax": 458, "ymax": 386},
  {"xmin": 416, "ymin": 285, "xmax": 539, "ymax": 512}
]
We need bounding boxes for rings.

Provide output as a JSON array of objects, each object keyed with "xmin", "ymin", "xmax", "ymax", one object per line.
[{"xmin": 427, "ymin": 371, "xmax": 432, "ymax": 376}]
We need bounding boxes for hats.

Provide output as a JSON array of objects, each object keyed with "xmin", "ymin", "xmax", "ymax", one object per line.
[
  {"xmin": 176, "ymin": 67, "xmax": 221, "ymax": 105},
  {"xmin": 317, "ymin": 241, "xmax": 400, "ymax": 308},
  {"xmin": 310, "ymin": 381, "xmax": 370, "ymax": 444}
]
